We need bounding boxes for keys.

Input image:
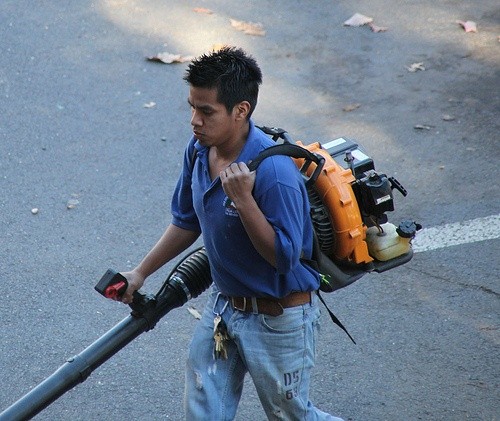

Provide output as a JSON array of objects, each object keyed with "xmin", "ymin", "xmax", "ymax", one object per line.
[{"xmin": 213, "ymin": 315, "xmax": 228, "ymax": 360}]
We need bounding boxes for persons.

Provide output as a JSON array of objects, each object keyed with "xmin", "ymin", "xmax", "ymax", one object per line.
[{"xmin": 119, "ymin": 50, "xmax": 338, "ymax": 420}]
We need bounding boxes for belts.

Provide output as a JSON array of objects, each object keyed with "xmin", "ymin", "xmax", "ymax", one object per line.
[{"xmin": 231, "ymin": 292, "xmax": 311, "ymax": 317}]
